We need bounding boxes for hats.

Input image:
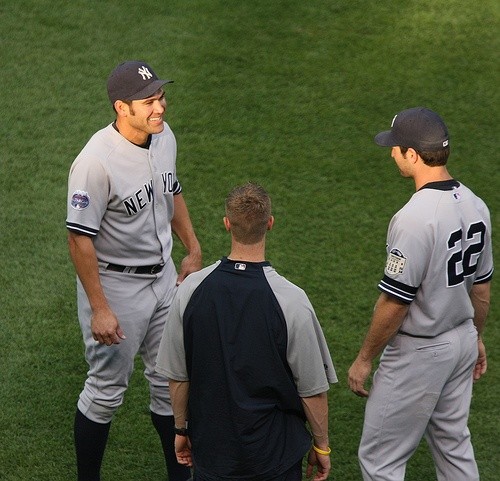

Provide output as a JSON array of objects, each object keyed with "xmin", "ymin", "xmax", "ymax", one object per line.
[
  {"xmin": 373, "ymin": 108, "xmax": 452, "ymax": 152},
  {"xmin": 107, "ymin": 61, "xmax": 176, "ymax": 108}
]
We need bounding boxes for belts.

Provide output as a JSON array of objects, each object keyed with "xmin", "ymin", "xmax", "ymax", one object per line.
[{"xmin": 105, "ymin": 262, "xmax": 165, "ymax": 275}]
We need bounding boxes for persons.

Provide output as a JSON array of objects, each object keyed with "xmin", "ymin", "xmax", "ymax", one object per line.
[
  {"xmin": 155, "ymin": 180, "xmax": 338, "ymax": 481},
  {"xmin": 346, "ymin": 106, "xmax": 494, "ymax": 481},
  {"xmin": 65, "ymin": 60, "xmax": 202, "ymax": 481}
]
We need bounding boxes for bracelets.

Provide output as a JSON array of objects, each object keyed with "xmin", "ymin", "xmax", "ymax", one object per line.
[
  {"xmin": 174, "ymin": 426, "xmax": 188, "ymax": 436},
  {"xmin": 311, "ymin": 444, "xmax": 331, "ymax": 456}
]
